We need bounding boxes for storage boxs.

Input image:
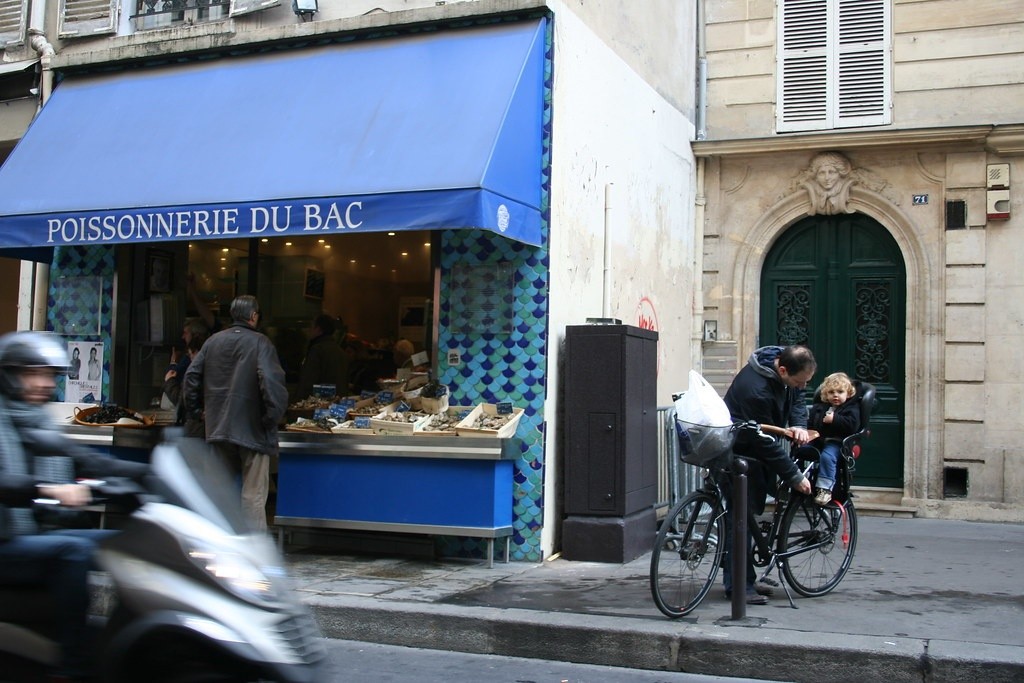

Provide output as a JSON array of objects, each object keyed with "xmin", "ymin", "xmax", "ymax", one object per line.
[{"xmin": 348, "ymin": 398, "xmax": 527, "ymax": 439}]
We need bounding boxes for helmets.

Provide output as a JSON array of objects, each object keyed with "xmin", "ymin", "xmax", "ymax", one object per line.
[{"xmin": 0, "ymin": 329, "xmax": 74, "ymax": 401}]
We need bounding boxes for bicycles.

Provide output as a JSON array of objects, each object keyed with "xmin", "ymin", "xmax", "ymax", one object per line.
[{"xmin": 649, "ymin": 379, "xmax": 877, "ymax": 619}]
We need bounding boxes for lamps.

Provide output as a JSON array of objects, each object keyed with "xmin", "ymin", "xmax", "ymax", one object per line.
[{"xmin": 291, "ymin": 0, "xmax": 319, "ymax": 22}]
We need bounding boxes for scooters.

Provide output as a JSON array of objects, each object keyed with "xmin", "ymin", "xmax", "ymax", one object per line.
[{"xmin": 0, "ymin": 431, "xmax": 344, "ymax": 683}]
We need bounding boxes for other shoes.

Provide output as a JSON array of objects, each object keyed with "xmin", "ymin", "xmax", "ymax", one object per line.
[
  {"xmin": 814, "ymin": 488, "xmax": 833, "ymax": 505},
  {"xmin": 723, "ymin": 585, "xmax": 769, "ymax": 604},
  {"xmin": 753, "ymin": 579, "xmax": 774, "ymax": 597}
]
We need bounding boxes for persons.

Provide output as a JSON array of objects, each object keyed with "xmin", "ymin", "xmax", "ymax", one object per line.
[
  {"xmin": 809, "ymin": 372, "xmax": 861, "ymax": 505},
  {"xmin": 807, "ymin": 152, "xmax": 851, "ymax": 215},
  {"xmin": 723, "ymin": 346, "xmax": 815, "ymax": 603},
  {"xmin": 277, "ymin": 314, "xmax": 376, "ymax": 403},
  {"xmin": 378, "ymin": 340, "xmax": 423, "ymax": 389},
  {"xmin": 0, "ymin": 331, "xmax": 163, "ymax": 683},
  {"xmin": 183, "ymin": 295, "xmax": 288, "ymax": 532},
  {"xmin": 164, "ymin": 317, "xmax": 210, "ymax": 426},
  {"xmin": 68, "ymin": 346, "xmax": 101, "ymax": 380}
]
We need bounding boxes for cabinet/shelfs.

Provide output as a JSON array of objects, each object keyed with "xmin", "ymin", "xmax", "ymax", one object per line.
[{"xmin": 564, "ymin": 325, "xmax": 659, "ymax": 514}]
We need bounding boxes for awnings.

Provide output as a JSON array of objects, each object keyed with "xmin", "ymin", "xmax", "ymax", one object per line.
[{"xmin": 0, "ymin": 19, "xmax": 543, "ymax": 250}]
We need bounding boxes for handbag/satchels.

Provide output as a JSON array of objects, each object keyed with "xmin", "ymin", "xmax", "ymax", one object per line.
[{"xmin": 676, "ymin": 370, "xmax": 735, "ymax": 464}]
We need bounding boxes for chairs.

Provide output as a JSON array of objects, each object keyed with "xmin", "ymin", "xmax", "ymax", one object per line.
[{"xmin": 809, "ymin": 378, "xmax": 877, "ymax": 508}]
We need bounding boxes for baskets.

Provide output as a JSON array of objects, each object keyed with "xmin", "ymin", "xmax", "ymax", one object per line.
[
  {"xmin": 673, "ymin": 414, "xmax": 744, "ymax": 470},
  {"xmin": 74, "ymin": 406, "xmax": 158, "ymax": 428}
]
